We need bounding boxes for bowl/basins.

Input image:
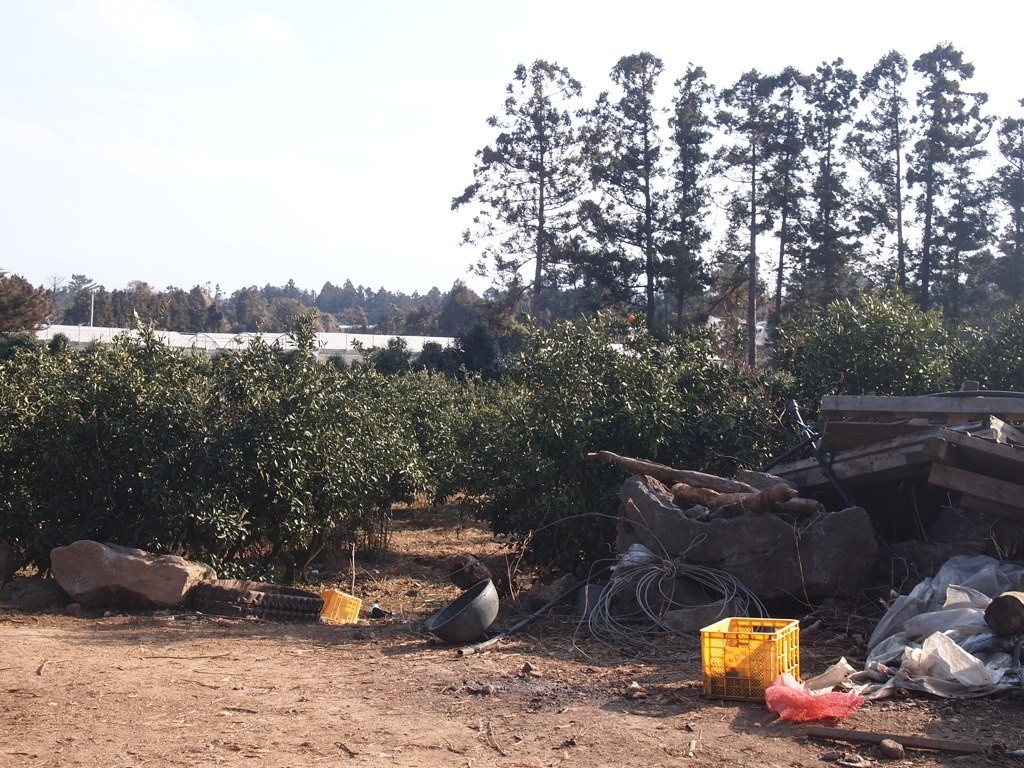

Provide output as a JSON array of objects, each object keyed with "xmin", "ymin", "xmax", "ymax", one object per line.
[{"xmin": 428, "ymin": 577, "xmax": 500, "ymax": 644}]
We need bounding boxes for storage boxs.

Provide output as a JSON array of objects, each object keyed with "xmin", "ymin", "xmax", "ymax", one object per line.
[
  {"xmin": 321, "ymin": 589, "xmax": 362, "ymax": 624},
  {"xmin": 699, "ymin": 617, "xmax": 800, "ymax": 700}
]
